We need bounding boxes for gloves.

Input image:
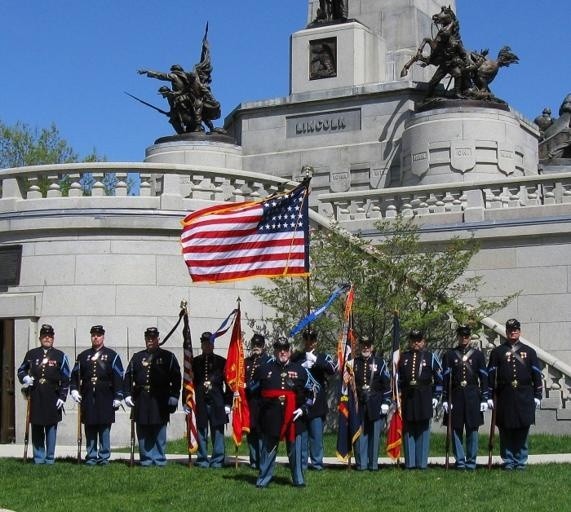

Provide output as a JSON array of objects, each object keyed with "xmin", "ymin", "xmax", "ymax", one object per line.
[
  {"xmin": 182, "ymin": 403, "xmax": 191, "ymax": 416},
  {"xmin": 224, "ymin": 405, "xmax": 232, "ymax": 415},
  {"xmin": 301, "ymin": 360, "xmax": 313, "ymax": 370},
  {"xmin": 442, "ymin": 401, "xmax": 453, "ymax": 413},
  {"xmin": 487, "ymin": 398, "xmax": 495, "ymax": 409},
  {"xmin": 124, "ymin": 396, "xmax": 135, "ymax": 408},
  {"xmin": 305, "ymin": 351, "xmax": 317, "ymax": 362},
  {"xmin": 168, "ymin": 396, "xmax": 179, "ymax": 406},
  {"xmin": 379, "ymin": 403, "xmax": 390, "ymax": 416},
  {"xmin": 22, "ymin": 375, "xmax": 34, "ymax": 387},
  {"xmin": 431, "ymin": 397, "xmax": 438, "ymax": 409},
  {"xmin": 533, "ymin": 397, "xmax": 542, "ymax": 408},
  {"xmin": 292, "ymin": 407, "xmax": 304, "ymax": 422},
  {"xmin": 479, "ymin": 401, "xmax": 488, "ymax": 412},
  {"xmin": 55, "ymin": 397, "xmax": 64, "ymax": 409},
  {"xmin": 71, "ymin": 389, "xmax": 82, "ymax": 404},
  {"xmin": 112, "ymin": 397, "xmax": 121, "ymax": 408}
]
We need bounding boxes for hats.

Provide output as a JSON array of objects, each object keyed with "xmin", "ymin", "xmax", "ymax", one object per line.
[
  {"xmin": 273, "ymin": 337, "xmax": 291, "ymax": 348},
  {"xmin": 410, "ymin": 328, "xmax": 424, "ymax": 338},
  {"xmin": 144, "ymin": 327, "xmax": 160, "ymax": 337},
  {"xmin": 39, "ymin": 324, "xmax": 55, "ymax": 336},
  {"xmin": 89, "ymin": 325, "xmax": 106, "ymax": 335},
  {"xmin": 303, "ymin": 328, "xmax": 319, "ymax": 337},
  {"xmin": 199, "ymin": 332, "xmax": 213, "ymax": 341},
  {"xmin": 456, "ymin": 326, "xmax": 472, "ymax": 336},
  {"xmin": 506, "ymin": 318, "xmax": 521, "ymax": 329},
  {"xmin": 359, "ymin": 333, "xmax": 374, "ymax": 344},
  {"xmin": 250, "ymin": 333, "xmax": 265, "ymax": 345}
]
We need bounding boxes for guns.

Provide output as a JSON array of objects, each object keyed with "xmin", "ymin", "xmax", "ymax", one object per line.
[
  {"xmin": 444, "ymin": 368, "xmax": 452, "ymax": 472},
  {"xmin": 487, "ymin": 367, "xmax": 498, "ymax": 472},
  {"xmin": 129, "ymin": 361, "xmax": 136, "ymax": 468},
  {"xmin": 23, "ymin": 361, "xmax": 32, "ymax": 467},
  {"xmin": 77, "ymin": 360, "xmax": 82, "ymax": 467}
]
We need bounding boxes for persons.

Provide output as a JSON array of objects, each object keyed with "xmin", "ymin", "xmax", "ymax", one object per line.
[
  {"xmin": 488, "ymin": 319, "xmax": 543, "ymax": 471},
  {"xmin": 442, "ymin": 326, "xmax": 488, "ymax": 473},
  {"xmin": 244, "ymin": 334, "xmax": 275, "ymax": 470},
  {"xmin": 70, "ymin": 325, "xmax": 124, "ymax": 466},
  {"xmin": 353, "ymin": 336, "xmax": 393, "ymax": 473},
  {"xmin": 397, "ymin": 329, "xmax": 442, "ymax": 472},
  {"xmin": 123, "ymin": 327, "xmax": 181, "ymax": 469},
  {"xmin": 290, "ymin": 329, "xmax": 336, "ymax": 471},
  {"xmin": 256, "ymin": 337, "xmax": 321, "ymax": 489},
  {"xmin": 136, "ymin": 40, "xmax": 222, "ymax": 132},
  {"xmin": 16, "ymin": 324, "xmax": 70, "ymax": 466},
  {"xmin": 192, "ymin": 332, "xmax": 233, "ymax": 469}
]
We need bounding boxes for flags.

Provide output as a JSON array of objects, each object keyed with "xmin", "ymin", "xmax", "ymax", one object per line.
[
  {"xmin": 180, "ymin": 178, "xmax": 310, "ymax": 285},
  {"xmin": 157, "ymin": 310, "xmax": 200, "ymax": 454},
  {"xmin": 290, "ymin": 282, "xmax": 363, "ymax": 462},
  {"xmin": 385, "ymin": 314, "xmax": 404, "ymax": 460},
  {"xmin": 210, "ymin": 308, "xmax": 251, "ymax": 448}
]
